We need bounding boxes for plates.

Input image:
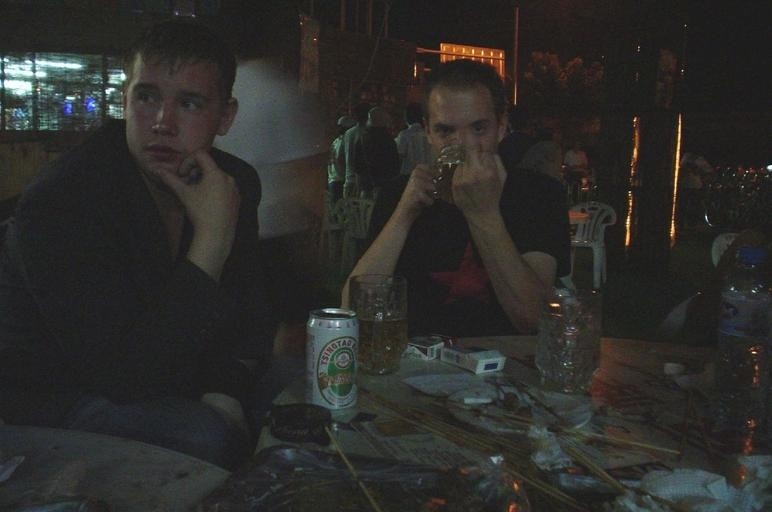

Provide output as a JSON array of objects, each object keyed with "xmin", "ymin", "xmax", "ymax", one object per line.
[{"xmin": 444, "ymin": 385, "xmax": 592, "ymax": 437}]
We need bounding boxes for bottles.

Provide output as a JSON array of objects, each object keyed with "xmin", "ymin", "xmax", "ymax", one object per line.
[{"xmin": 701, "ymin": 245, "xmax": 772, "ymax": 448}]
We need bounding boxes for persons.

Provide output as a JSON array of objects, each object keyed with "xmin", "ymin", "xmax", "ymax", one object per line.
[
  {"xmin": 311, "ymin": 96, "xmax": 595, "ymax": 200},
  {"xmin": 0, "ymin": 12, "xmax": 298, "ymax": 487},
  {"xmin": 340, "ymin": 58, "xmax": 575, "ymax": 344}
]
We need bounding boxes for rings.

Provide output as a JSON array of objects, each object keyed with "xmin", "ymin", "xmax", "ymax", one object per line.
[{"xmin": 200, "ymin": 162, "xmax": 218, "ymax": 176}]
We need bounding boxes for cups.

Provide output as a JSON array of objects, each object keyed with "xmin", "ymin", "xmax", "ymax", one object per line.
[
  {"xmin": 348, "ymin": 273, "xmax": 409, "ymax": 378},
  {"xmin": 534, "ymin": 285, "xmax": 603, "ymax": 396},
  {"xmin": 431, "ymin": 143, "xmax": 469, "ymax": 207}
]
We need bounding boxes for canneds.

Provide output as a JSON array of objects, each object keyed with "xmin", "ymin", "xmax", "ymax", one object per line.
[{"xmin": 305, "ymin": 308, "xmax": 361, "ymax": 413}]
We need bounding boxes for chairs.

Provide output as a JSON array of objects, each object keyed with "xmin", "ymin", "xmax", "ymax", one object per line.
[
  {"xmin": 318, "ymin": 182, "xmax": 375, "ymax": 281},
  {"xmin": 567, "ymin": 200, "xmax": 617, "ymax": 288}
]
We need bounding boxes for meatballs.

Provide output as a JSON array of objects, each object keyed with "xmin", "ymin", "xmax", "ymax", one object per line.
[{"xmin": 503, "ymin": 391, "xmax": 532, "ymax": 421}]
